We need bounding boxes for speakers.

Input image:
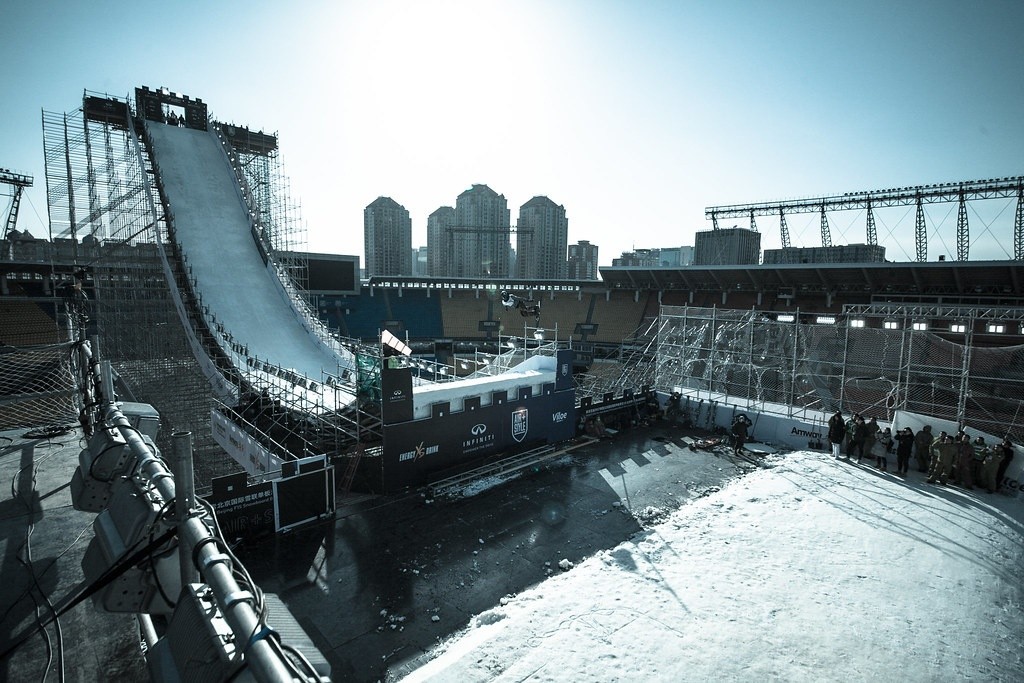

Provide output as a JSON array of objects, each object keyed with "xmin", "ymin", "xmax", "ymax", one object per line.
[{"xmin": 71, "ymin": 401, "xmax": 331, "ymax": 683}]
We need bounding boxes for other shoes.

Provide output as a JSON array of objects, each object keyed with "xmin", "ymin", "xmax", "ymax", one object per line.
[
  {"xmin": 995, "ymin": 488, "xmax": 1000, "ymax": 492},
  {"xmin": 953, "ymin": 481, "xmax": 962, "ymax": 485},
  {"xmin": 875, "ymin": 464, "xmax": 881, "ymax": 469},
  {"xmin": 735, "ymin": 448, "xmax": 741, "ymax": 455},
  {"xmin": 918, "ymin": 467, "xmax": 928, "ymax": 472},
  {"xmin": 964, "ymin": 485, "xmax": 974, "ymax": 490},
  {"xmin": 940, "ymin": 480, "xmax": 947, "ymax": 486},
  {"xmin": 927, "ymin": 477, "xmax": 936, "ymax": 483},
  {"xmin": 857, "ymin": 459, "xmax": 862, "ymax": 464},
  {"xmin": 986, "ymin": 488, "xmax": 994, "ymax": 494},
  {"xmin": 927, "ymin": 473, "xmax": 932, "ymax": 477},
  {"xmin": 895, "ymin": 470, "xmax": 906, "ymax": 477},
  {"xmin": 844, "ymin": 457, "xmax": 850, "ymax": 462},
  {"xmin": 830, "ymin": 454, "xmax": 839, "ymax": 460},
  {"xmin": 880, "ymin": 467, "xmax": 886, "ymax": 470}
]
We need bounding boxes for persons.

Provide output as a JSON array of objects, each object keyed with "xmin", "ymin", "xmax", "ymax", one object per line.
[
  {"xmin": 926, "ymin": 435, "xmax": 959, "ymax": 485},
  {"xmin": 585, "ymin": 414, "xmax": 613, "ymax": 441},
  {"xmin": 826, "ymin": 413, "xmax": 845, "ymax": 460},
  {"xmin": 952, "ymin": 430, "xmax": 965, "ymax": 449},
  {"xmin": 731, "ymin": 416, "xmax": 748, "ymax": 457},
  {"xmin": 845, "ymin": 414, "xmax": 860, "ymax": 457},
  {"xmin": 982, "ymin": 444, "xmax": 1005, "ymax": 493},
  {"xmin": 840, "ymin": 416, "xmax": 867, "ymax": 463},
  {"xmin": 926, "ymin": 430, "xmax": 947, "ymax": 477},
  {"xmin": 663, "ymin": 391, "xmax": 683, "ymax": 413},
  {"xmin": 953, "ymin": 434, "xmax": 975, "ymax": 491},
  {"xmin": 827, "ymin": 411, "xmax": 845, "ymax": 453},
  {"xmin": 871, "ymin": 427, "xmax": 891, "ymax": 470},
  {"xmin": 993, "ymin": 440, "xmax": 1014, "ymax": 490},
  {"xmin": 913, "ymin": 424, "xmax": 934, "ymax": 473},
  {"xmin": 892, "ymin": 426, "xmax": 914, "ymax": 477},
  {"xmin": 864, "ymin": 416, "xmax": 879, "ymax": 460},
  {"xmin": 498, "ymin": 291, "xmax": 541, "ymax": 321},
  {"xmin": 971, "ymin": 436, "xmax": 988, "ymax": 477}
]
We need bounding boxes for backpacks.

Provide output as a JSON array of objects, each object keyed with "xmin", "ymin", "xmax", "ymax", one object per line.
[{"xmin": 887, "ymin": 437, "xmax": 894, "ymax": 454}]
